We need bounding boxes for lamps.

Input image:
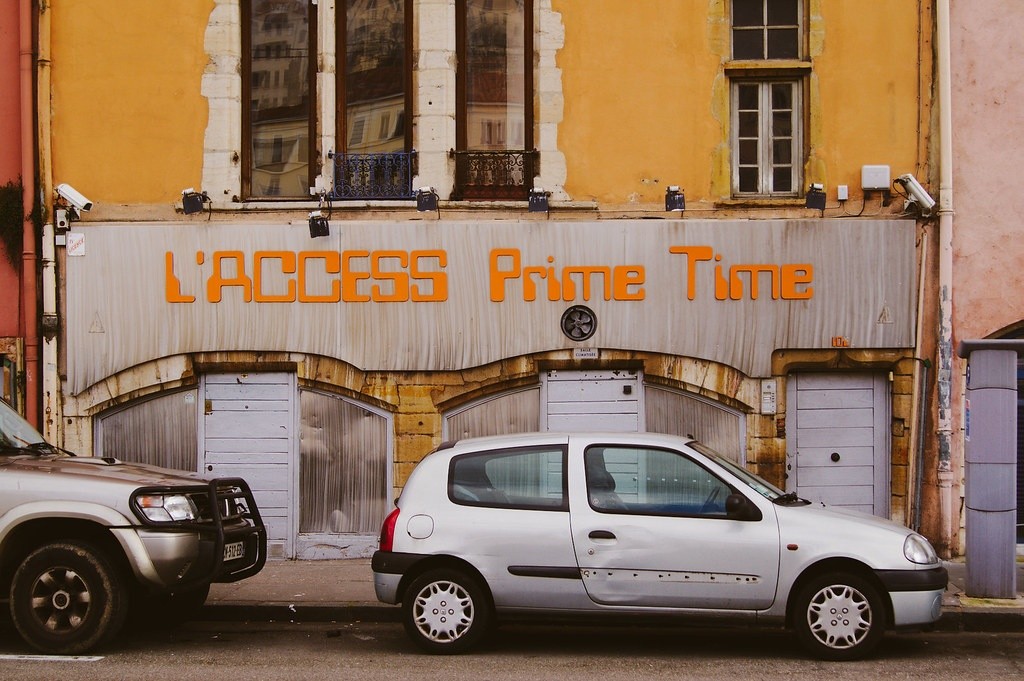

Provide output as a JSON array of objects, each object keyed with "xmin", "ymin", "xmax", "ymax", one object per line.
[
  {"xmin": 182, "ymin": 193, "xmax": 202, "ymax": 214},
  {"xmin": 805, "ymin": 186, "xmax": 828, "ymax": 211},
  {"xmin": 664, "ymin": 185, "xmax": 685, "ymax": 211},
  {"xmin": 308, "ymin": 215, "xmax": 329, "ymax": 238}
]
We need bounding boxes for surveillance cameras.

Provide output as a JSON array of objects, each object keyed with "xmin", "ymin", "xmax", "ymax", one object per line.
[
  {"xmin": 57, "ymin": 183, "xmax": 92, "ymax": 212},
  {"xmin": 900, "ymin": 174, "xmax": 935, "ymax": 210}
]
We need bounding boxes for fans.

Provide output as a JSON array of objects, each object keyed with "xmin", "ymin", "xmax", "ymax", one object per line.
[{"xmin": 560, "ymin": 305, "xmax": 598, "ymax": 341}]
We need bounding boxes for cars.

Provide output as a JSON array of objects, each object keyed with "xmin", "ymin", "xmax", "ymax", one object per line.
[{"xmin": 372, "ymin": 429, "xmax": 949, "ymax": 661}]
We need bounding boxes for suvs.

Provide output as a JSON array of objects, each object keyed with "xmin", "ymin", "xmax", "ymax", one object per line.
[{"xmin": 0, "ymin": 398, "xmax": 269, "ymax": 656}]
